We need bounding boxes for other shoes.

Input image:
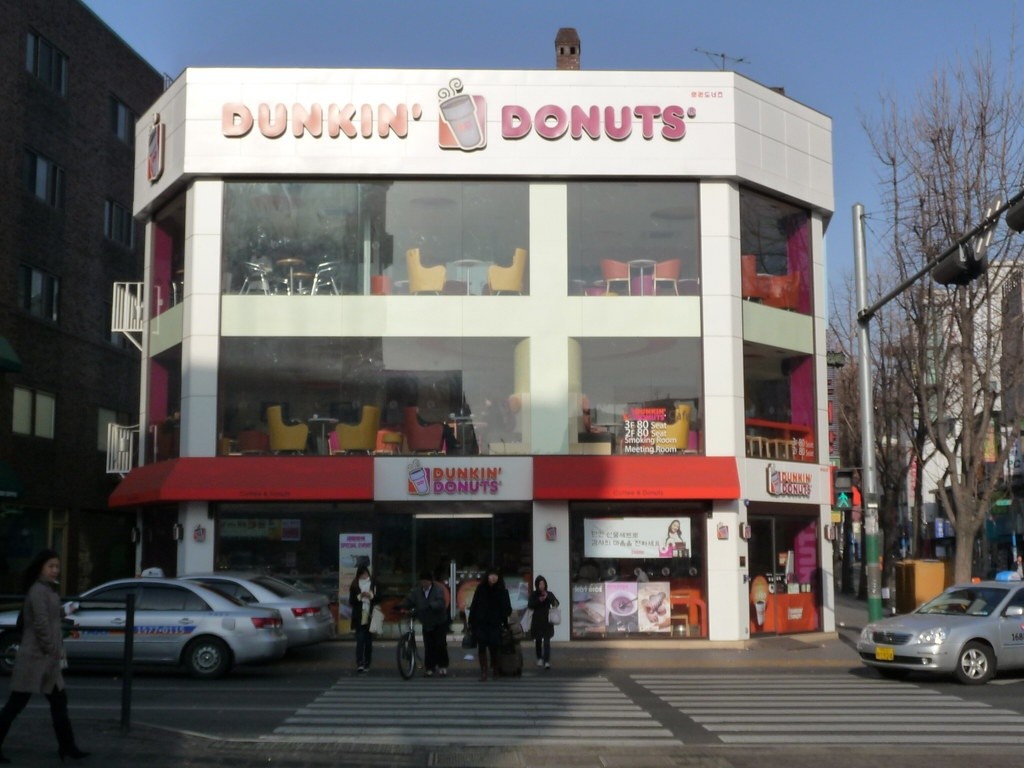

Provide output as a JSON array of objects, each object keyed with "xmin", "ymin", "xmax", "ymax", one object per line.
[
  {"xmin": 478, "ymin": 672, "xmax": 488, "ymax": 682},
  {"xmin": 492, "ymin": 671, "xmax": 498, "ymax": 680},
  {"xmin": 357, "ymin": 663, "xmax": 364, "ymax": 674},
  {"xmin": 440, "ymin": 667, "xmax": 447, "ymax": 677},
  {"xmin": 537, "ymin": 658, "xmax": 543, "ymax": 666},
  {"xmin": 364, "ymin": 662, "xmax": 372, "ymax": 673},
  {"xmin": 425, "ymin": 669, "xmax": 433, "ymax": 676},
  {"xmin": 544, "ymin": 662, "xmax": 551, "ymax": 669}
]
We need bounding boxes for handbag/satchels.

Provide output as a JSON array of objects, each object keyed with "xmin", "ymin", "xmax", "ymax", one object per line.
[
  {"xmin": 547, "ymin": 591, "xmax": 560, "ymax": 625},
  {"xmin": 462, "ymin": 627, "xmax": 477, "ymax": 649},
  {"xmin": 519, "ymin": 591, "xmax": 534, "ymax": 634},
  {"xmin": 369, "ymin": 608, "xmax": 385, "ymax": 635},
  {"xmin": 501, "ymin": 623, "xmax": 516, "ymax": 656}
]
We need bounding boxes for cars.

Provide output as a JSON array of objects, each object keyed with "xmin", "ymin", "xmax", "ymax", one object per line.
[
  {"xmin": 855, "ymin": 571, "xmax": 1024, "ymax": 685},
  {"xmin": 173, "ymin": 572, "xmax": 337, "ymax": 658},
  {"xmin": 0, "ymin": 567, "xmax": 288, "ymax": 683}
]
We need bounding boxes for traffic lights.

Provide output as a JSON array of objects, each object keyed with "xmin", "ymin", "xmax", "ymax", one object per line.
[{"xmin": 835, "ymin": 468, "xmax": 857, "ymax": 511}]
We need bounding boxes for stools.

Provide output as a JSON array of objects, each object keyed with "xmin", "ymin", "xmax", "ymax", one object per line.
[
  {"xmin": 371, "ymin": 276, "xmax": 392, "ymax": 295},
  {"xmin": 671, "ymin": 614, "xmax": 691, "ymax": 636},
  {"xmin": 744, "ymin": 435, "xmax": 802, "ymax": 461},
  {"xmin": 382, "ymin": 432, "xmax": 401, "ymax": 456},
  {"xmin": 240, "ymin": 431, "xmax": 268, "ymax": 455},
  {"xmin": 218, "ymin": 438, "xmax": 243, "ymax": 456}
]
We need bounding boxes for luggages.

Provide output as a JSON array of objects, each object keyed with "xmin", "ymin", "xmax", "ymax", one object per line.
[{"xmin": 500, "ymin": 630, "xmax": 523, "ymax": 678}]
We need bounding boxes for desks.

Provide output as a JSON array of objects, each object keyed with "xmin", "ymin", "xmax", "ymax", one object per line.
[
  {"xmin": 451, "ymin": 416, "xmax": 486, "ymax": 454},
  {"xmin": 277, "ymin": 259, "xmax": 305, "ymax": 295},
  {"xmin": 450, "ymin": 259, "xmax": 483, "ymax": 295},
  {"xmin": 745, "ymin": 417, "xmax": 809, "ymax": 439},
  {"xmin": 626, "ymin": 259, "xmax": 656, "ymax": 296},
  {"xmin": 293, "ymin": 273, "xmax": 312, "ymax": 295},
  {"xmin": 309, "ymin": 418, "xmax": 339, "ymax": 456},
  {"xmin": 594, "ymin": 423, "xmax": 623, "ymax": 432}
]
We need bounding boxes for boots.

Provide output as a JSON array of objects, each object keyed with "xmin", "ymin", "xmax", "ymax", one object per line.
[
  {"xmin": 0, "ymin": 719, "xmax": 11, "ymax": 763},
  {"xmin": 54, "ymin": 719, "xmax": 91, "ymax": 762}
]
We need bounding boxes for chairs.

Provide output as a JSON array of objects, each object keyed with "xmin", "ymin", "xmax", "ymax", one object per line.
[
  {"xmin": 266, "ymin": 403, "xmax": 517, "ymax": 455},
  {"xmin": 572, "ymin": 259, "xmax": 700, "ymax": 296},
  {"xmin": 405, "ymin": 247, "xmax": 528, "ymax": 295},
  {"xmin": 741, "ymin": 255, "xmax": 800, "ymax": 312},
  {"xmin": 582, "ymin": 398, "xmax": 698, "ymax": 454},
  {"xmin": 238, "ymin": 261, "xmax": 342, "ymax": 295}
]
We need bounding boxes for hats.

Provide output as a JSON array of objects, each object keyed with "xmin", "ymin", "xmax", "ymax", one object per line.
[{"xmin": 416, "ymin": 568, "xmax": 435, "ymax": 582}]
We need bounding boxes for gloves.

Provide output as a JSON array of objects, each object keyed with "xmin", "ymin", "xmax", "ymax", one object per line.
[
  {"xmin": 418, "ymin": 605, "xmax": 431, "ymax": 611},
  {"xmin": 392, "ymin": 604, "xmax": 403, "ymax": 610}
]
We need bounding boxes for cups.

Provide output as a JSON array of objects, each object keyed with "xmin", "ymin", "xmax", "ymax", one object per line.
[
  {"xmin": 755, "ymin": 601, "xmax": 765, "ymax": 626},
  {"xmin": 606, "ymin": 590, "xmax": 638, "ymax": 616}
]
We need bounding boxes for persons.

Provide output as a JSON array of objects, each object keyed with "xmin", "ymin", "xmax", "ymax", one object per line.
[
  {"xmin": 349, "ymin": 565, "xmax": 378, "ymax": 673},
  {"xmin": 1011, "ymin": 554, "xmax": 1024, "ymax": 580},
  {"xmin": 468, "ymin": 566, "xmax": 513, "ymax": 682},
  {"xmin": 0, "ymin": 548, "xmax": 93, "ymax": 760},
  {"xmin": 407, "ymin": 401, "xmax": 458, "ymax": 453},
  {"xmin": 663, "ymin": 520, "xmax": 686, "ymax": 557},
  {"xmin": 527, "ymin": 575, "xmax": 559, "ymax": 671},
  {"xmin": 470, "ymin": 395, "xmax": 505, "ymax": 455},
  {"xmin": 633, "ymin": 562, "xmax": 649, "ymax": 582},
  {"xmin": 959, "ymin": 591, "xmax": 976, "ymax": 611},
  {"xmin": 389, "ymin": 571, "xmax": 450, "ymax": 678}
]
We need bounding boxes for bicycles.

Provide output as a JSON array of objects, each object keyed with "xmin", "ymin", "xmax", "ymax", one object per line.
[{"xmin": 392, "ymin": 609, "xmax": 424, "ymax": 681}]
